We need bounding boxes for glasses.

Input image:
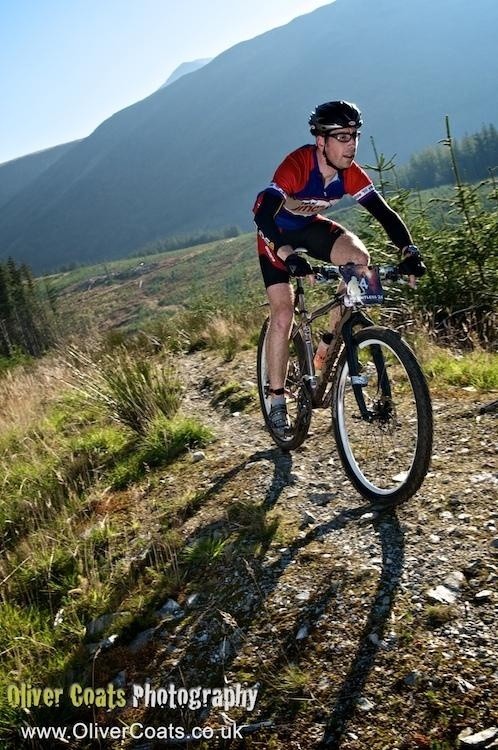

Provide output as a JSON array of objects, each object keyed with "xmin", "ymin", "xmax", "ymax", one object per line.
[{"xmin": 327, "ymin": 131, "xmax": 361, "ymax": 143}]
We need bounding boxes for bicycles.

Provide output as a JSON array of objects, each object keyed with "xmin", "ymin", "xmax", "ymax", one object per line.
[{"xmin": 256, "ymin": 261, "xmax": 432, "ymax": 506}]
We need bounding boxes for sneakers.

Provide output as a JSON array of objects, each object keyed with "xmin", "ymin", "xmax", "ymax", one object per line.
[
  {"xmin": 268, "ymin": 400, "xmax": 291, "ymax": 436},
  {"xmin": 293, "ymin": 379, "xmax": 327, "ymax": 402}
]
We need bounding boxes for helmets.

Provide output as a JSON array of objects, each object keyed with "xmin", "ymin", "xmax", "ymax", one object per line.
[{"xmin": 308, "ymin": 100, "xmax": 364, "ymax": 136}]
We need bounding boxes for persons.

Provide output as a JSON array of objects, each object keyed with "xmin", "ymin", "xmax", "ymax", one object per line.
[{"xmin": 253, "ymin": 100, "xmax": 427, "ymax": 432}]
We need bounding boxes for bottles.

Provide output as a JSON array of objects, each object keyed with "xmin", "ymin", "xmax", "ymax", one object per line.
[{"xmin": 313, "ymin": 332, "xmax": 335, "ymax": 370}]
